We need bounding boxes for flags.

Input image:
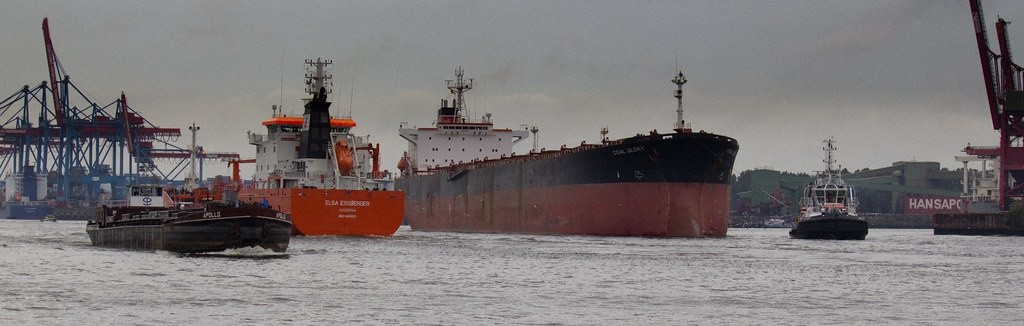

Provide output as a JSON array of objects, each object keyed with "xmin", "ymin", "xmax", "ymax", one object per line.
[
  {"xmin": 163, "ymin": 191, "xmax": 173, "ymax": 209},
  {"xmin": 260, "ymin": 193, "xmax": 268, "ymax": 209}
]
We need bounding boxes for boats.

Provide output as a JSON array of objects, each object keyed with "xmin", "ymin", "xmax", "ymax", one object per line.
[
  {"xmin": 85, "ymin": 183, "xmax": 292, "ymax": 258},
  {"xmin": 787, "ymin": 133, "xmax": 869, "ymax": 241},
  {"xmin": 161, "ymin": 56, "xmax": 405, "ymax": 238},
  {"xmin": 42, "ymin": 215, "xmax": 57, "ymax": 225},
  {"xmin": 395, "ymin": 62, "xmax": 740, "ymax": 236}
]
